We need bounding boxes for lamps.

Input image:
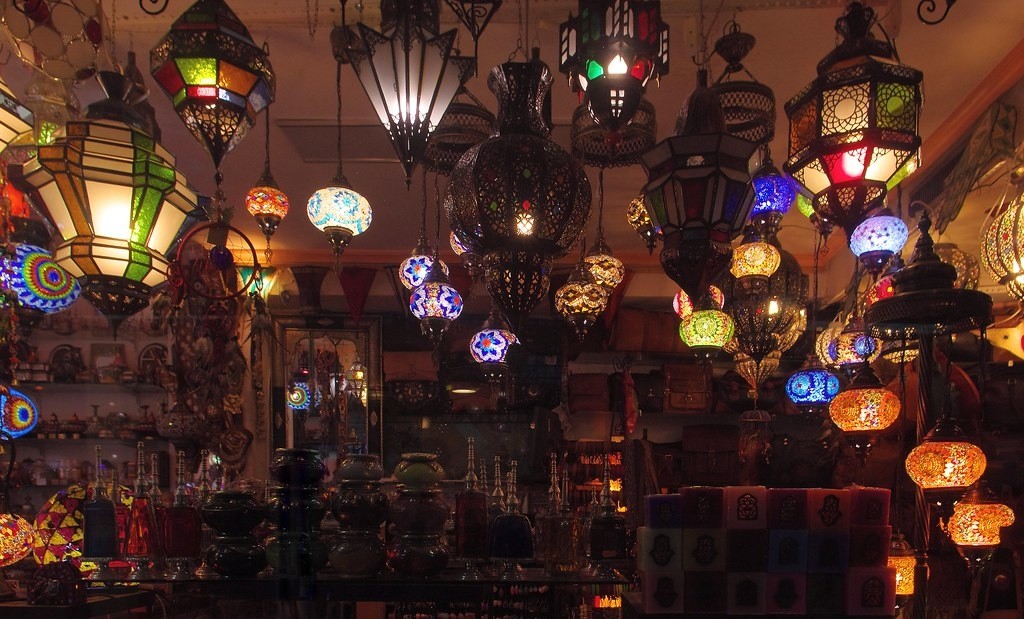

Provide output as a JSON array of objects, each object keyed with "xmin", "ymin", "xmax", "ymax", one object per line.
[{"xmin": 0, "ymin": 0, "xmax": 1024, "ymax": 603}]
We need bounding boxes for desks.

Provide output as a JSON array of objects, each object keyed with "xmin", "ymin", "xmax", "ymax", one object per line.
[
  {"xmin": 0, "ymin": 591, "xmax": 155, "ymax": 619},
  {"xmin": 77, "ymin": 562, "xmax": 631, "ymax": 619}
]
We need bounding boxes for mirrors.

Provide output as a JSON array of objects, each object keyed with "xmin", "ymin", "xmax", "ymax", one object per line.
[{"xmin": 270, "ymin": 314, "xmax": 383, "ymax": 484}]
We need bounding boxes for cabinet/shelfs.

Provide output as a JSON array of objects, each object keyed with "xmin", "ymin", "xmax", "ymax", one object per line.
[
  {"xmin": 0, "ymin": 381, "xmax": 169, "ymax": 489},
  {"xmin": 570, "ymin": 348, "xmax": 917, "ymax": 506}
]
